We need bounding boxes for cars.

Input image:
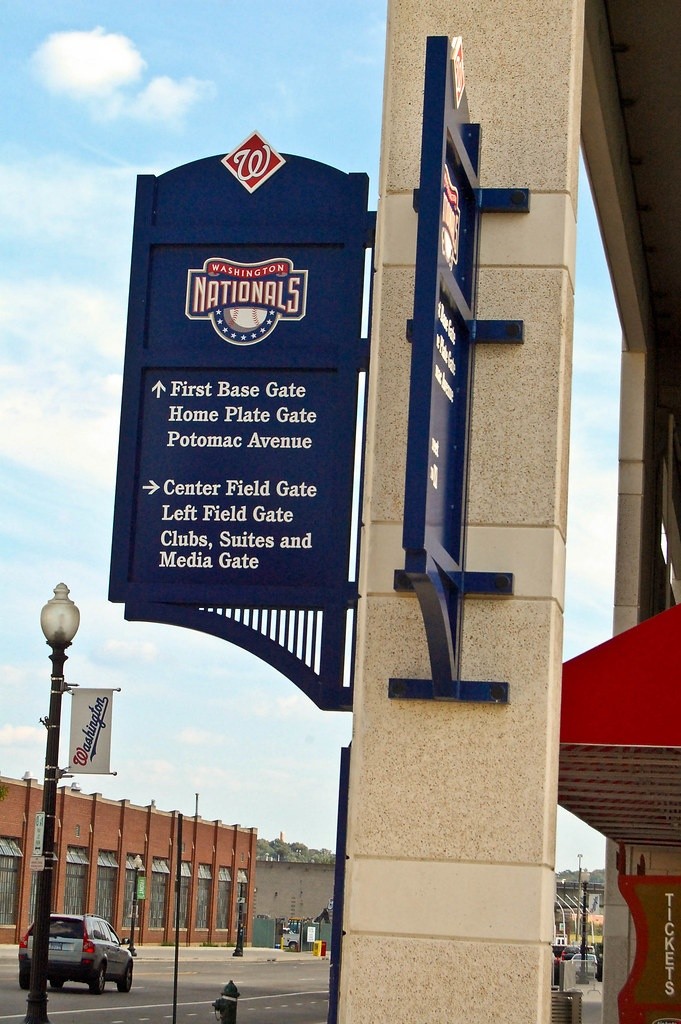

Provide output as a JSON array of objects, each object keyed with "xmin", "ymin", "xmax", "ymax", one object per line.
[{"xmin": 553, "ymin": 944, "xmax": 603, "ymax": 985}]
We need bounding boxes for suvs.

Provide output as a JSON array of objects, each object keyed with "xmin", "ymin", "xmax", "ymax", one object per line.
[{"xmin": 18, "ymin": 912, "xmax": 135, "ymax": 994}]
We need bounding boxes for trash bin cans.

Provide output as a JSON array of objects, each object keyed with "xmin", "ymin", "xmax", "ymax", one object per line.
[{"xmin": 552, "ymin": 991, "xmax": 583, "ymax": 1024}]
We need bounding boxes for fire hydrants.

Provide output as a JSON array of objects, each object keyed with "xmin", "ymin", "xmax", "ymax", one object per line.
[{"xmin": 211, "ymin": 980, "xmax": 243, "ymax": 1024}]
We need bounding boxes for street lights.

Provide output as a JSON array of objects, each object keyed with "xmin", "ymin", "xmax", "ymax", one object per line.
[
  {"xmin": 125, "ymin": 855, "xmax": 143, "ymax": 957},
  {"xmin": 234, "ymin": 869, "xmax": 248, "ymax": 956},
  {"xmin": 25, "ymin": 580, "xmax": 82, "ymax": 1024},
  {"xmin": 575, "ymin": 868, "xmax": 591, "ymax": 984}
]
